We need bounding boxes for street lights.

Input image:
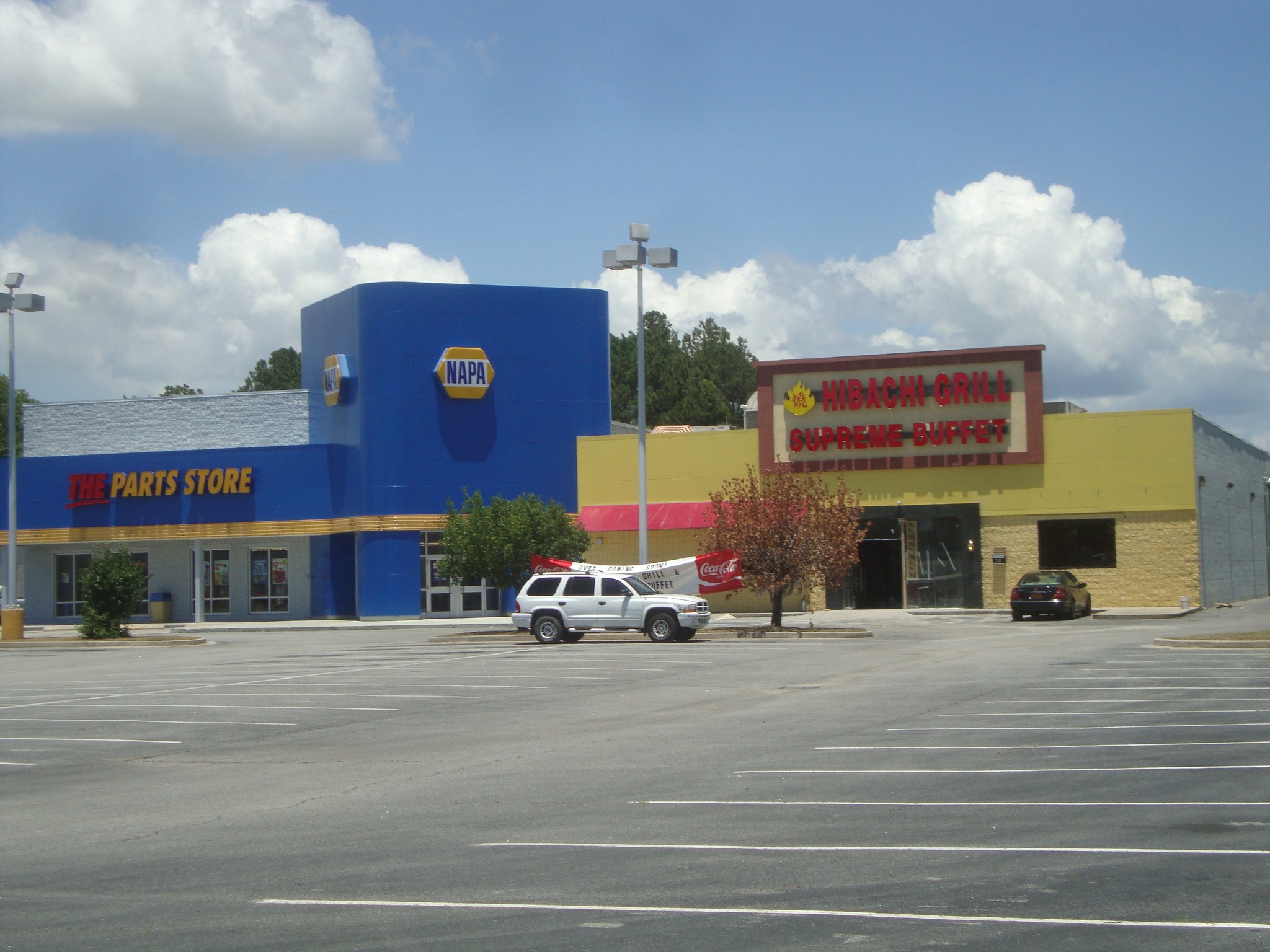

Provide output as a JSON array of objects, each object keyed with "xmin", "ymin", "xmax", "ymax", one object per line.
[
  {"xmin": 0, "ymin": 271, "xmax": 45, "ymax": 641},
  {"xmin": 601, "ymin": 222, "xmax": 677, "ymax": 564}
]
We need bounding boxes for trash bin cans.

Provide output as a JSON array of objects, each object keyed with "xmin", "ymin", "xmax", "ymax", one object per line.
[{"xmin": 150, "ymin": 591, "xmax": 172, "ymax": 622}]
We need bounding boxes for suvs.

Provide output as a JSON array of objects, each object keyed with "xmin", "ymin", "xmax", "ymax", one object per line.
[{"xmin": 512, "ymin": 570, "xmax": 710, "ymax": 643}]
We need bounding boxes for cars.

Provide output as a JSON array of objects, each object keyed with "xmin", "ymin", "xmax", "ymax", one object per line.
[{"xmin": 1010, "ymin": 569, "xmax": 1091, "ymax": 619}]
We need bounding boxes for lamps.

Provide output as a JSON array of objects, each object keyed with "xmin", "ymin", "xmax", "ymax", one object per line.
[{"xmin": 967, "ymin": 540, "xmax": 975, "ymax": 552}]
